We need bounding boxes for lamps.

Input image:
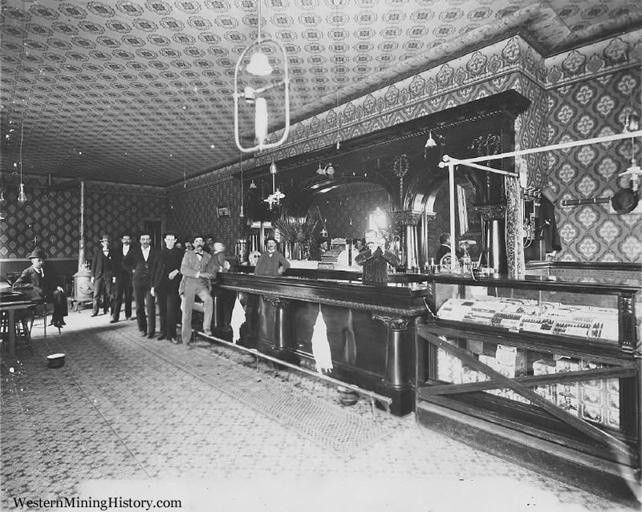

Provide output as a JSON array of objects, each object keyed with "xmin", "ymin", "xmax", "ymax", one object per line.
[
  {"xmin": 423, "ymin": 128, "xmax": 438, "ymax": 150},
  {"xmin": 619, "ymin": 114, "xmax": 641, "ymax": 191},
  {"xmin": 17, "ymin": 107, "xmax": 28, "ymax": 203},
  {"xmin": 264, "ymin": 158, "xmax": 285, "ymax": 210},
  {"xmin": 244, "ymin": 0, "xmax": 275, "ymax": 80}
]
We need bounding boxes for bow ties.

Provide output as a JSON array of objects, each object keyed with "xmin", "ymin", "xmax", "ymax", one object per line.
[{"xmin": 194, "ymin": 252, "xmax": 204, "ymax": 259}]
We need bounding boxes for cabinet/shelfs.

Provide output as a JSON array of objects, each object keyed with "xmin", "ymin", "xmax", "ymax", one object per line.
[{"xmin": 415, "ymin": 264, "xmax": 642, "ymax": 477}]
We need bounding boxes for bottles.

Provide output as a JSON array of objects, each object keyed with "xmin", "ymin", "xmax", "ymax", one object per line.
[{"xmin": 298, "ymin": 245, "xmax": 313, "ymax": 261}]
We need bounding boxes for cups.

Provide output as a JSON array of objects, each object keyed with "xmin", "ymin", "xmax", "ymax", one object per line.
[{"xmin": 433, "ymin": 264, "xmax": 440, "ymax": 274}]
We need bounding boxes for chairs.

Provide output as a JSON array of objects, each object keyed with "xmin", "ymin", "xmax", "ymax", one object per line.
[{"xmin": 14, "ymin": 271, "xmax": 63, "ymax": 337}]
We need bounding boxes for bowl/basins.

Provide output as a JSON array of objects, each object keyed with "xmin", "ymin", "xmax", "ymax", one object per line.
[{"xmin": 47, "ymin": 353, "xmax": 66, "ymax": 367}]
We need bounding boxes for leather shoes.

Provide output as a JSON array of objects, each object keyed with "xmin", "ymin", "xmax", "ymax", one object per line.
[
  {"xmin": 140, "ymin": 330, "xmax": 193, "ymax": 350},
  {"xmin": 51, "ymin": 315, "xmax": 66, "ymax": 329},
  {"xmin": 90, "ymin": 309, "xmax": 98, "ymax": 317},
  {"xmin": 125, "ymin": 315, "xmax": 134, "ymax": 320},
  {"xmin": 111, "ymin": 315, "xmax": 120, "ymax": 324},
  {"xmin": 102, "ymin": 307, "xmax": 108, "ymax": 315},
  {"xmin": 203, "ymin": 327, "xmax": 213, "ymax": 336}
]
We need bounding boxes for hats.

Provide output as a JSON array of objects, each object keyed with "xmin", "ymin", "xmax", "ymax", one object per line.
[
  {"xmin": 100, "ymin": 235, "xmax": 112, "ymax": 243},
  {"xmin": 264, "ymin": 236, "xmax": 278, "ymax": 247},
  {"xmin": 28, "ymin": 249, "xmax": 48, "ymax": 259}
]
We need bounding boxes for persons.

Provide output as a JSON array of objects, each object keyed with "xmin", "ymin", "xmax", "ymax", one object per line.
[
  {"xmin": 11, "ymin": 232, "xmax": 290, "ymax": 348},
  {"xmin": 436, "ymin": 231, "xmax": 462, "ymax": 264},
  {"xmin": 355, "ymin": 229, "xmax": 401, "ymax": 283}
]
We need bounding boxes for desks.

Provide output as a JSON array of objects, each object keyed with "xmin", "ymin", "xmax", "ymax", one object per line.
[{"xmin": 0, "ymin": 278, "xmax": 32, "ymax": 356}]
